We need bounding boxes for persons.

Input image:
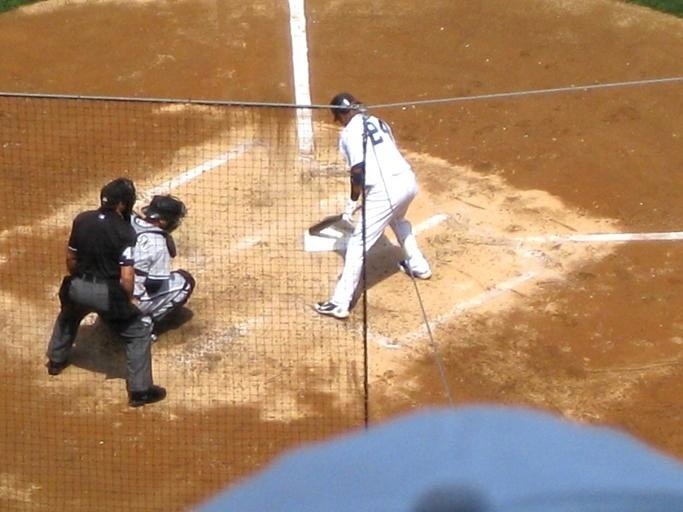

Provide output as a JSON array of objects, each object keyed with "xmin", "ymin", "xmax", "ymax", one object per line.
[
  {"xmin": 45, "ymin": 177, "xmax": 167, "ymax": 407},
  {"xmin": 309, "ymin": 92, "xmax": 432, "ymax": 319},
  {"xmin": 118, "ymin": 195, "xmax": 197, "ymax": 341}
]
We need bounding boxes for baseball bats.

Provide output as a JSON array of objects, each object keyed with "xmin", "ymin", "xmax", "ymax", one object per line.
[{"xmin": 309, "ymin": 205, "xmax": 362, "ymax": 235}]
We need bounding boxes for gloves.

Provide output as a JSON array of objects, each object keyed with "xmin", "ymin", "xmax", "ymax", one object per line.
[{"xmin": 342, "ymin": 198, "xmax": 359, "ymax": 220}]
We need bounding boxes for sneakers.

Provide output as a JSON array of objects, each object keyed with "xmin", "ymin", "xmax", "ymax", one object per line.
[
  {"xmin": 313, "ymin": 299, "xmax": 350, "ymax": 319},
  {"xmin": 128, "ymin": 384, "xmax": 167, "ymax": 408},
  {"xmin": 141, "ymin": 316, "xmax": 160, "ymax": 342},
  {"xmin": 397, "ymin": 260, "xmax": 433, "ymax": 280},
  {"xmin": 46, "ymin": 359, "xmax": 72, "ymax": 375}
]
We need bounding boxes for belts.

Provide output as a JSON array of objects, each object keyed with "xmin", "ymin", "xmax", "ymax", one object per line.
[{"xmin": 75, "ymin": 271, "xmax": 108, "ymax": 285}]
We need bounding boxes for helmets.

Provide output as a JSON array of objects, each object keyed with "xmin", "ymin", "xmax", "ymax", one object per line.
[
  {"xmin": 142, "ymin": 194, "xmax": 186, "ymax": 231},
  {"xmin": 329, "ymin": 91, "xmax": 360, "ymax": 120},
  {"xmin": 100, "ymin": 178, "xmax": 138, "ymax": 219}
]
